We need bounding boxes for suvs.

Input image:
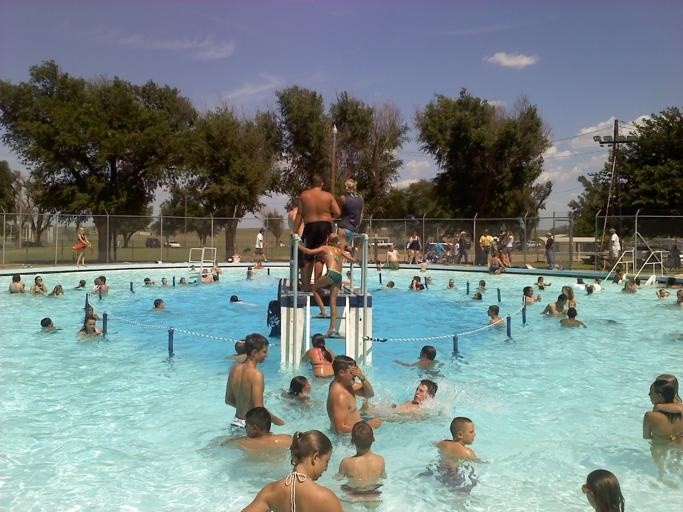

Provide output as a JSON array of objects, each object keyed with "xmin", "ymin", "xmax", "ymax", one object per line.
[{"xmin": 145, "ymin": 237, "xmax": 161, "ymax": 248}]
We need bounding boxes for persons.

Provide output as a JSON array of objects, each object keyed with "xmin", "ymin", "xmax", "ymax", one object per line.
[
  {"xmin": 338, "ymin": 179, "xmax": 364, "ymax": 252},
  {"xmin": 607, "ymin": 228, "xmax": 624, "ymax": 272},
  {"xmin": 582, "ymin": 469, "xmax": 624, "ymax": 512},
  {"xmin": 40, "ymin": 318, "xmax": 54, "ymax": 330},
  {"xmin": 537, "ymin": 277, "xmax": 551, "ymax": 289},
  {"xmin": 542, "ymin": 294, "xmax": 567, "ymax": 317},
  {"xmin": 411, "ymin": 276, "xmax": 432, "ymax": 290},
  {"xmin": 291, "ymin": 175, "xmax": 341, "ymax": 292},
  {"xmin": 386, "ymin": 245, "xmax": 401, "ymax": 270},
  {"xmin": 242, "ymin": 431, "xmax": 343, "ymax": 512},
  {"xmin": 387, "ymin": 281, "xmax": 394, "ymax": 288},
  {"xmin": 359, "ymin": 379, "xmax": 438, "ymax": 418},
  {"xmin": 342, "ymin": 271, "xmax": 351, "ymax": 286},
  {"xmin": 569, "ymin": 299, "xmax": 577, "ymax": 311},
  {"xmin": 487, "ymin": 305, "xmax": 503, "ymax": 324},
  {"xmin": 77, "ymin": 227, "xmax": 92, "ymax": 265},
  {"xmin": 523, "ymin": 286, "xmax": 539, "ymax": 304},
  {"xmin": 326, "ymin": 355, "xmax": 382, "ymax": 435},
  {"xmin": 643, "ymin": 380, "xmax": 683, "ymax": 439},
  {"xmin": 225, "ymin": 334, "xmax": 286, "ymax": 429},
  {"xmin": 221, "ymin": 406, "xmax": 293, "ymax": 451},
  {"xmin": 92, "ymin": 276, "xmax": 109, "ymax": 293},
  {"xmin": 447, "ymin": 279, "xmax": 457, "ymax": 290},
  {"xmin": 246, "ymin": 264, "xmax": 263, "ymax": 280},
  {"xmin": 30, "ymin": 276, "xmax": 48, "ymax": 293},
  {"xmin": 394, "ymin": 345, "xmax": 435, "ymax": 369},
  {"xmin": 376, "ymin": 260, "xmax": 382, "ymax": 271},
  {"xmin": 48, "ymin": 285, "xmax": 63, "ymax": 296},
  {"xmin": 230, "ymin": 295, "xmax": 238, "ymax": 302},
  {"xmin": 281, "ymin": 376, "xmax": 311, "ymax": 405},
  {"xmin": 154, "ymin": 299, "xmax": 164, "ymax": 309},
  {"xmin": 654, "ymin": 375, "xmax": 683, "ymax": 413},
  {"xmin": 577, "ymin": 276, "xmax": 602, "ymax": 295},
  {"xmin": 144, "ymin": 261, "xmax": 222, "ymax": 287},
  {"xmin": 288, "ymin": 199, "xmax": 304, "ymax": 268},
  {"xmin": 302, "ymin": 334, "xmax": 335, "ymax": 378},
  {"xmin": 472, "ymin": 293, "xmax": 483, "ymax": 300},
  {"xmin": 267, "ymin": 301, "xmax": 281, "ymax": 338},
  {"xmin": 9, "ymin": 274, "xmax": 25, "ymax": 293},
  {"xmin": 75, "ymin": 280, "xmax": 86, "ymax": 289},
  {"xmin": 665, "ymin": 244, "xmax": 681, "ymax": 270},
  {"xmin": 79, "ymin": 318, "xmax": 101, "ymax": 338},
  {"xmin": 560, "ymin": 308, "xmax": 580, "ymax": 328},
  {"xmin": 232, "ymin": 340, "xmax": 247, "ymax": 362},
  {"xmin": 433, "ymin": 417, "xmax": 483, "ymax": 468},
  {"xmin": 332, "ymin": 423, "xmax": 385, "ymax": 489},
  {"xmin": 407, "ymin": 228, "xmax": 513, "ymax": 275},
  {"xmin": 545, "ymin": 233, "xmax": 554, "ymax": 270},
  {"xmin": 88, "ymin": 305, "xmax": 99, "ymax": 321},
  {"xmin": 298, "ymin": 233, "xmax": 359, "ymax": 338},
  {"xmin": 562, "ymin": 285, "xmax": 576, "ymax": 308},
  {"xmin": 251, "ymin": 227, "xmax": 270, "ymax": 262},
  {"xmin": 479, "ymin": 280, "xmax": 486, "ymax": 289},
  {"xmin": 613, "ymin": 269, "xmax": 683, "ymax": 307}
]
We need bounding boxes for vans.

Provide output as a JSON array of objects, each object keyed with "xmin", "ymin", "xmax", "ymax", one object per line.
[{"xmin": 527, "ymin": 241, "xmax": 538, "ymax": 248}]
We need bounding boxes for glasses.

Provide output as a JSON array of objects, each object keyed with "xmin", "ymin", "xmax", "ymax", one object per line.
[{"xmin": 581, "ymin": 485, "xmax": 591, "ymax": 494}]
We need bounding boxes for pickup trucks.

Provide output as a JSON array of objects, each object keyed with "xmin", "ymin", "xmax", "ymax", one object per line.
[{"xmin": 369, "ymin": 239, "xmax": 394, "ymax": 248}]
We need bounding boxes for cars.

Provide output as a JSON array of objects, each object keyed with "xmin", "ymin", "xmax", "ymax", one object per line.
[{"xmin": 165, "ymin": 240, "xmax": 181, "ymax": 248}]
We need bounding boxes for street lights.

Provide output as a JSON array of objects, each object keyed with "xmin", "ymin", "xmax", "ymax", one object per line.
[
  {"xmin": 330, "ymin": 124, "xmax": 338, "ymax": 195},
  {"xmin": 593, "ymin": 119, "xmax": 638, "ymax": 262}
]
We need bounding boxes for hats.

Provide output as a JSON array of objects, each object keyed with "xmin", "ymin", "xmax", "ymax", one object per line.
[
  {"xmin": 545, "ymin": 232, "xmax": 551, "ymax": 236},
  {"xmin": 608, "ymin": 229, "xmax": 616, "ymax": 232}
]
